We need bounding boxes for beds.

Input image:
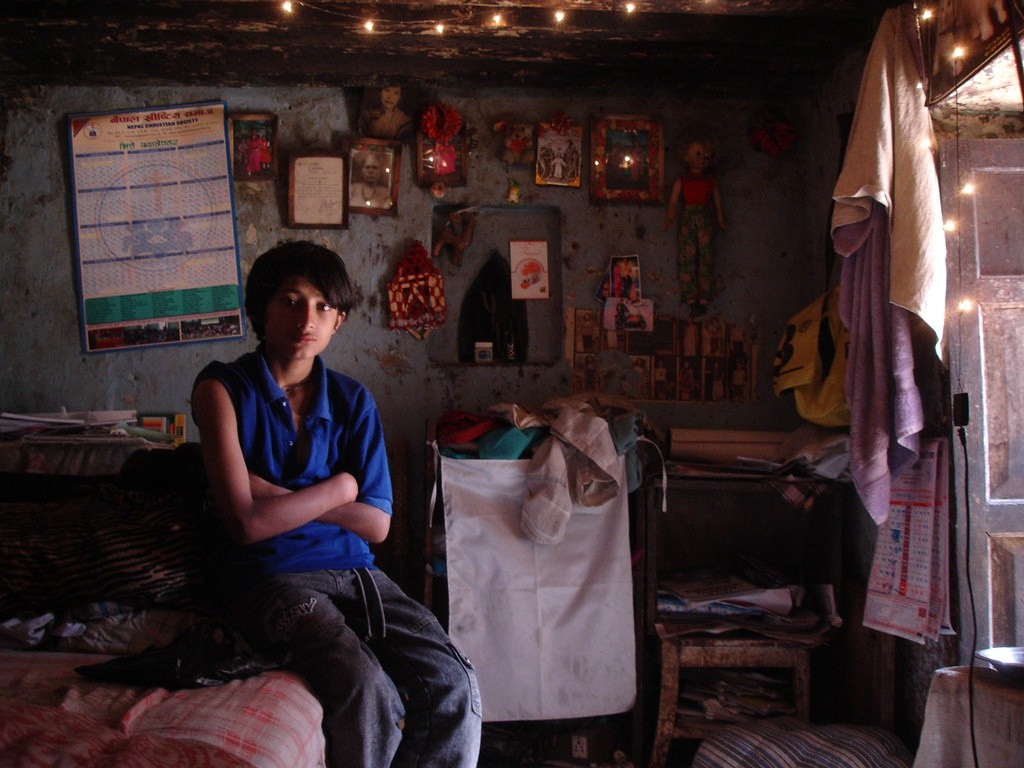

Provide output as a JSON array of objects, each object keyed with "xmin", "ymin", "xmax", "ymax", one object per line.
[{"xmin": 0, "ymin": 502, "xmax": 330, "ymax": 768}]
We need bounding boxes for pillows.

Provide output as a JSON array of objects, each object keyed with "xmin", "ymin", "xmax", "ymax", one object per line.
[{"xmin": 0, "ymin": 480, "xmax": 212, "ymax": 614}]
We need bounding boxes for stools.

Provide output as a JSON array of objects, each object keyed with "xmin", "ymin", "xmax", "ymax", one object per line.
[{"xmin": 649, "ymin": 639, "xmax": 811, "ymax": 768}]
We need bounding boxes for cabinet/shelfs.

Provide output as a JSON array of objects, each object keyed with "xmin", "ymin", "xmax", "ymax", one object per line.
[{"xmin": 422, "ymin": 418, "xmax": 646, "ymax": 768}]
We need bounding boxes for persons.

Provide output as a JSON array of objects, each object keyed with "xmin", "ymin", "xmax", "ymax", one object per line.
[
  {"xmin": 191, "ymin": 239, "xmax": 483, "ymax": 768},
  {"xmin": 667, "ymin": 126, "xmax": 726, "ymax": 317},
  {"xmin": 232, "ymin": 74, "xmax": 755, "ymax": 403}
]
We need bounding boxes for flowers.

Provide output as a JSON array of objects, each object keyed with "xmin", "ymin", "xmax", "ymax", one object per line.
[{"xmin": 421, "ymin": 102, "xmax": 463, "ymax": 144}]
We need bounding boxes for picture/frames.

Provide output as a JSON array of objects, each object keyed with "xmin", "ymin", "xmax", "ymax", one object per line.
[
  {"xmin": 590, "ymin": 113, "xmax": 665, "ymax": 208},
  {"xmin": 534, "ymin": 123, "xmax": 585, "ymax": 188},
  {"xmin": 342, "ymin": 136, "xmax": 403, "ymax": 217},
  {"xmin": 287, "ymin": 150, "xmax": 348, "ymax": 230},
  {"xmin": 416, "ymin": 121, "xmax": 468, "ymax": 188},
  {"xmin": 227, "ymin": 113, "xmax": 279, "ymax": 181}
]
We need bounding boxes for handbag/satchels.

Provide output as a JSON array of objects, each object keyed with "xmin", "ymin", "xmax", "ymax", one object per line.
[{"xmin": 770, "ymin": 286, "xmax": 851, "ymax": 428}]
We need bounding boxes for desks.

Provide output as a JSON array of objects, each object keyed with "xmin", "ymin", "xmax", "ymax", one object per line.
[
  {"xmin": 911, "ymin": 665, "xmax": 1024, "ymax": 768},
  {"xmin": 643, "ymin": 471, "xmax": 846, "ymax": 638}
]
